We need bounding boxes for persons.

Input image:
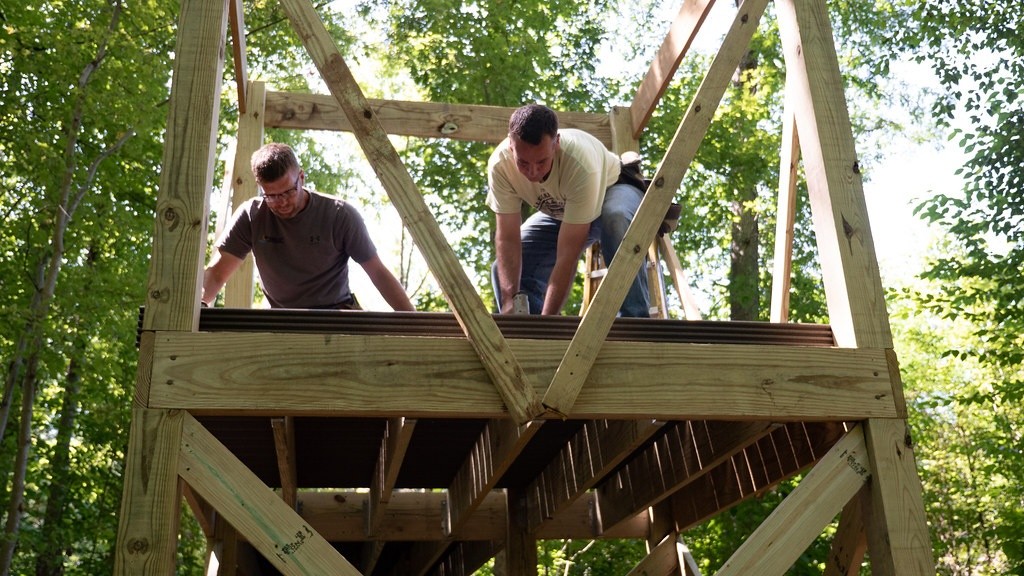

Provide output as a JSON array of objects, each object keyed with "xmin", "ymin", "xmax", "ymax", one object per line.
[
  {"xmin": 484, "ymin": 104, "xmax": 652, "ymax": 318},
  {"xmin": 200, "ymin": 142, "xmax": 416, "ymax": 311}
]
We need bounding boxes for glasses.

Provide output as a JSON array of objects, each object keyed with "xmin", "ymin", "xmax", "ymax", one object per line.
[{"xmin": 261, "ymin": 175, "xmax": 300, "ymax": 202}]
